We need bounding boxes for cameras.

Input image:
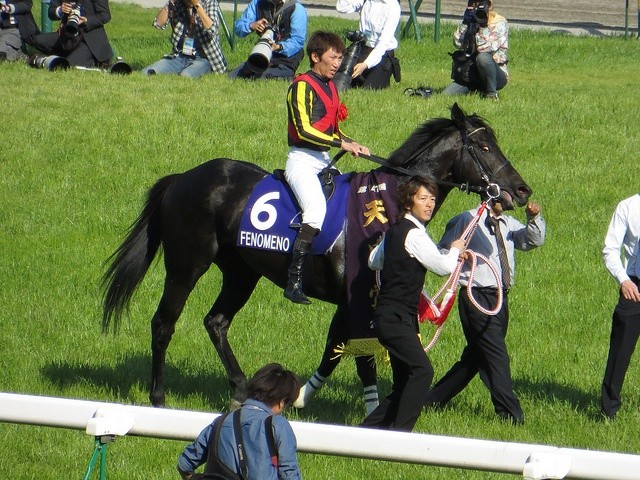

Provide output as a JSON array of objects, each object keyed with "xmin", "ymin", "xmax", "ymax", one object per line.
[
  {"xmin": 28, "ymin": 53, "xmax": 70, "ymax": 72},
  {"xmin": 342, "ymin": 25, "xmax": 364, "ymax": 91},
  {"xmin": 168, "ymin": 0, "xmax": 195, "ymax": 21},
  {"xmin": 463, "ymin": 0, "xmax": 493, "ymax": 27},
  {"xmin": 67, "ymin": 2, "xmax": 80, "ymax": 35},
  {"xmin": 247, "ymin": 18, "xmax": 278, "ymax": 74}
]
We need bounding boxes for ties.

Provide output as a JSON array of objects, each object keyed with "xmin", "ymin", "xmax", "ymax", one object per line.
[{"xmin": 491, "ymin": 216, "xmax": 511, "ymax": 289}]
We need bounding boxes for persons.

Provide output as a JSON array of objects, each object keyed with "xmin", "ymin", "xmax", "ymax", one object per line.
[
  {"xmin": 177, "ymin": 363, "xmax": 302, "ymax": 480},
  {"xmin": 335, "ymin": 0, "xmax": 402, "ymax": 90},
  {"xmin": 140, "ymin": 0, "xmax": 227, "ymax": 77},
  {"xmin": 440, "ymin": 0, "xmax": 509, "ymax": 101},
  {"xmin": 226, "ymin": 0, "xmax": 308, "ymax": 80},
  {"xmin": 283, "ymin": 31, "xmax": 371, "ymax": 304},
  {"xmin": 359, "ymin": 176, "xmax": 469, "ymax": 432},
  {"xmin": 29, "ymin": 0, "xmax": 113, "ymax": 69},
  {"xmin": 601, "ymin": 194, "xmax": 640, "ymax": 417},
  {"xmin": 0, "ymin": 0, "xmax": 40, "ymax": 64},
  {"xmin": 425, "ymin": 194, "xmax": 545, "ymax": 424}
]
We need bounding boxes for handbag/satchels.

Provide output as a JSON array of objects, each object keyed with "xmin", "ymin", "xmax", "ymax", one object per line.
[
  {"xmin": 447, "ymin": 45, "xmax": 477, "ymax": 87},
  {"xmin": 190, "ymin": 410, "xmax": 241, "ymax": 480}
]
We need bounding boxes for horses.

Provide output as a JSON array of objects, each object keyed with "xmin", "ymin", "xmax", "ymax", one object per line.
[{"xmin": 98, "ymin": 103, "xmax": 532, "ymax": 412}]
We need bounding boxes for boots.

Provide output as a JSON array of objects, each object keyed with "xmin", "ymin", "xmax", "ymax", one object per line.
[{"xmin": 283, "ymin": 223, "xmax": 320, "ymax": 304}]
